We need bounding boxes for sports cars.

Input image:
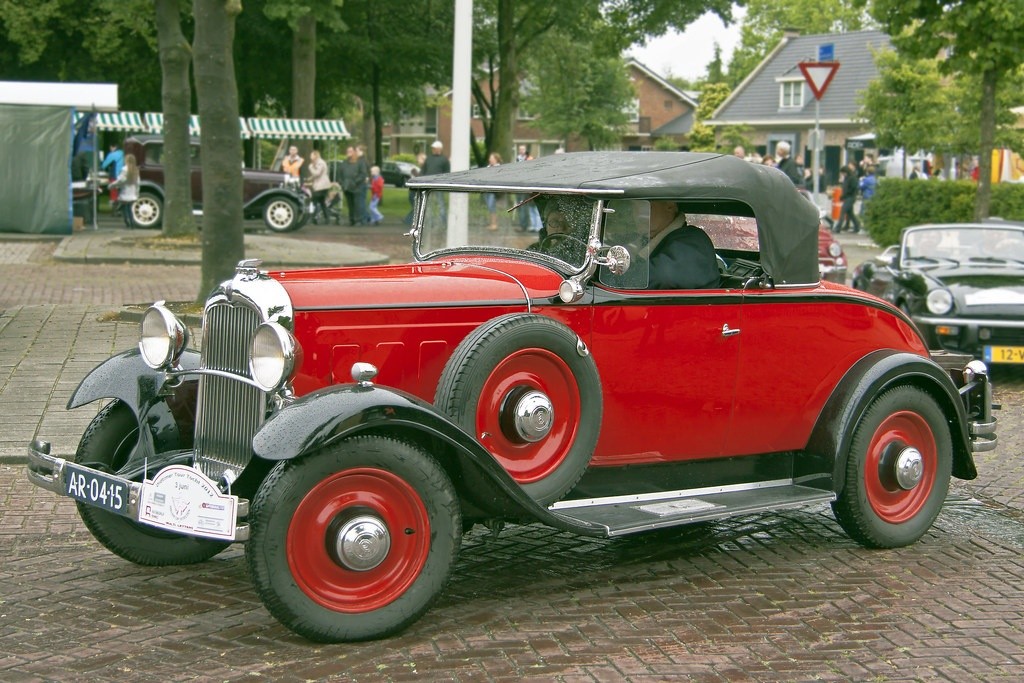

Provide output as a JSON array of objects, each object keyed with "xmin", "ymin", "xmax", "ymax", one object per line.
[{"xmin": 853, "ymin": 217, "xmax": 1024, "ymax": 377}]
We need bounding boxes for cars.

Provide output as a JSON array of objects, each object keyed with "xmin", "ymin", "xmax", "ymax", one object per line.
[
  {"xmin": 121, "ymin": 135, "xmax": 315, "ymax": 232},
  {"xmin": 380, "ymin": 162, "xmax": 423, "ymax": 188},
  {"xmin": 627, "ymin": 186, "xmax": 847, "ymax": 287},
  {"xmin": 0, "ymin": 150, "xmax": 1002, "ymax": 641},
  {"xmin": 327, "ymin": 159, "xmax": 344, "ymax": 181}
]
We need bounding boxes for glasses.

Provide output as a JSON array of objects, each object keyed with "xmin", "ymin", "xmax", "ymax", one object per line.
[{"xmin": 546, "ymin": 218, "xmax": 575, "ymax": 228}]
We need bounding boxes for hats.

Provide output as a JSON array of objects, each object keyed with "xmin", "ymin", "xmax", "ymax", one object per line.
[{"xmin": 431, "ymin": 142, "xmax": 442, "ymax": 148}]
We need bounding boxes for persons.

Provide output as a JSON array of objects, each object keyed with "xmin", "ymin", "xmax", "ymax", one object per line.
[
  {"xmin": 336, "ymin": 146, "xmax": 367, "ymax": 225},
  {"xmin": 514, "ymin": 143, "xmax": 544, "ymax": 232},
  {"xmin": 302, "ymin": 151, "xmax": 331, "ymax": 225},
  {"xmin": 366, "ymin": 165, "xmax": 384, "ymax": 224},
  {"xmin": 401, "ymin": 140, "xmax": 450, "ymax": 229},
  {"xmin": 835, "ymin": 154, "xmax": 881, "ymax": 236},
  {"xmin": 412, "ymin": 144, "xmax": 427, "ymax": 167},
  {"xmin": 528, "ymin": 196, "xmax": 590, "ymax": 265},
  {"xmin": 484, "ymin": 151, "xmax": 507, "ymax": 230},
  {"xmin": 107, "ymin": 152, "xmax": 140, "ymax": 229},
  {"xmin": 282, "ymin": 145, "xmax": 304, "ymax": 178},
  {"xmin": 355, "ymin": 144, "xmax": 370, "ymax": 222},
  {"xmin": 101, "ymin": 137, "xmax": 126, "ymax": 217},
  {"xmin": 733, "ymin": 141, "xmax": 827, "ymax": 193},
  {"xmin": 612, "ymin": 198, "xmax": 721, "ymax": 290}
]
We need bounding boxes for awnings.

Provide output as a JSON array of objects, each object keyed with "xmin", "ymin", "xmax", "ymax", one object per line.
[{"xmin": 845, "ymin": 130, "xmax": 878, "ymax": 149}]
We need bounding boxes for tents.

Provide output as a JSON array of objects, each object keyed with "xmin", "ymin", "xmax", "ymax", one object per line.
[
  {"xmin": 70, "ymin": 110, "xmax": 145, "ymax": 227},
  {"xmin": 248, "ymin": 116, "xmax": 351, "ymax": 171},
  {"xmin": 142, "ymin": 111, "xmax": 251, "ymax": 139}
]
